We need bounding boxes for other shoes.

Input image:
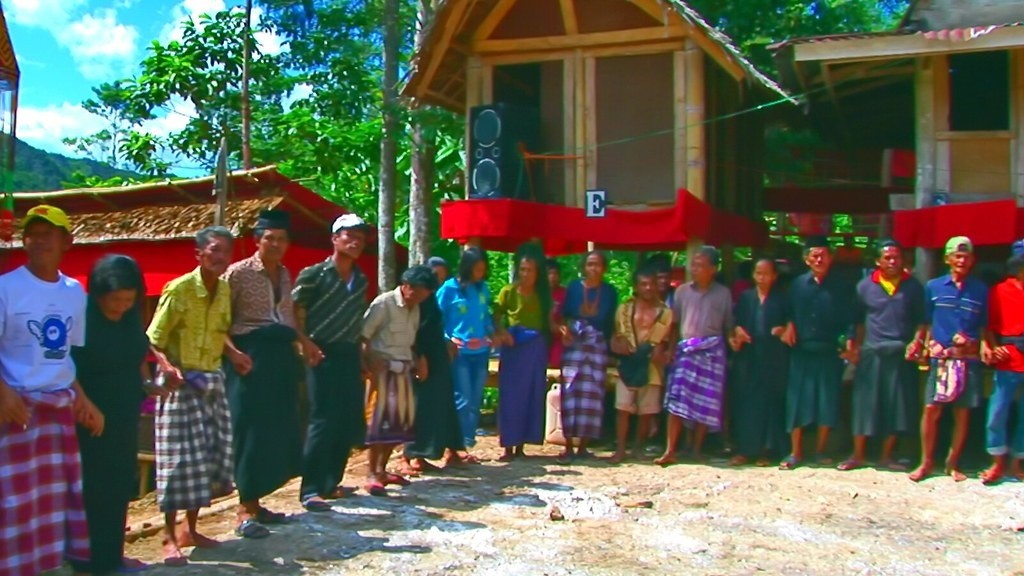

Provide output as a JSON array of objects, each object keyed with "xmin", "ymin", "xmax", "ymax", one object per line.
[{"xmin": 496, "ymin": 444, "xmax": 1024, "ymax": 484}]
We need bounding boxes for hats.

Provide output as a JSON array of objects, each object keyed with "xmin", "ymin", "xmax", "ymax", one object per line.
[
  {"xmin": 945, "ymin": 237, "xmax": 973, "ymax": 256},
  {"xmin": 19, "ymin": 204, "xmax": 70, "ymax": 233},
  {"xmin": 332, "ymin": 213, "xmax": 366, "ymax": 233},
  {"xmin": 255, "ymin": 207, "xmax": 291, "ymax": 230}
]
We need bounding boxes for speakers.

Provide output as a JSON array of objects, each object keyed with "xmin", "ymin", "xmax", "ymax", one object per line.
[{"xmin": 467, "ymin": 100, "xmax": 546, "ymax": 205}]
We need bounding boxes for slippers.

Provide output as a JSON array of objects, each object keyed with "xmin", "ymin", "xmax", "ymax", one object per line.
[
  {"xmin": 444, "ymin": 455, "xmax": 467, "ymax": 467},
  {"xmin": 235, "ymin": 518, "xmax": 270, "ymax": 538},
  {"xmin": 321, "ymin": 486, "xmax": 360, "ymax": 499},
  {"xmin": 386, "ymin": 474, "xmax": 410, "ymax": 484},
  {"xmin": 365, "ymin": 480, "xmax": 385, "ymax": 495},
  {"xmin": 414, "ymin": 461, "xmax": 439, "ymax": 471},
  {"xmin": 256, "ymin": 507, "xmax": 286, "ymax": 522},
  {"xmin": 301, "ymin": 493, "xmax": 331, "ymax": 512}
]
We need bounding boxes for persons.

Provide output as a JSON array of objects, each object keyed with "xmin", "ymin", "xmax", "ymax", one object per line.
[
  {"xmin": 0, "ymin": 207, "xmax": 1024, "ymax": 576},
  {"xmin": 0, "ymin": 206, "xmax": 106, "ymax": 576},
  {"xmin": 148, "ymin": 226, "xmax": 254, "ymax": 566},
  {"xmin": 80, "ymin": 254, "xmax": 171, "ymax": 576}
]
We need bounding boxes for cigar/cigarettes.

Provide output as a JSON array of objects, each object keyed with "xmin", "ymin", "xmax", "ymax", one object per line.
[{"xmin": 24, "ymin": 423, "xmax": 27, "ymax": 431}]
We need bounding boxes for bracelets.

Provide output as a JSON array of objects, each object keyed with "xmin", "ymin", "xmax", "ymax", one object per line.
[{"xmin": 141, "ymin": 378, "xmax": 151, "ymax": 386}]
